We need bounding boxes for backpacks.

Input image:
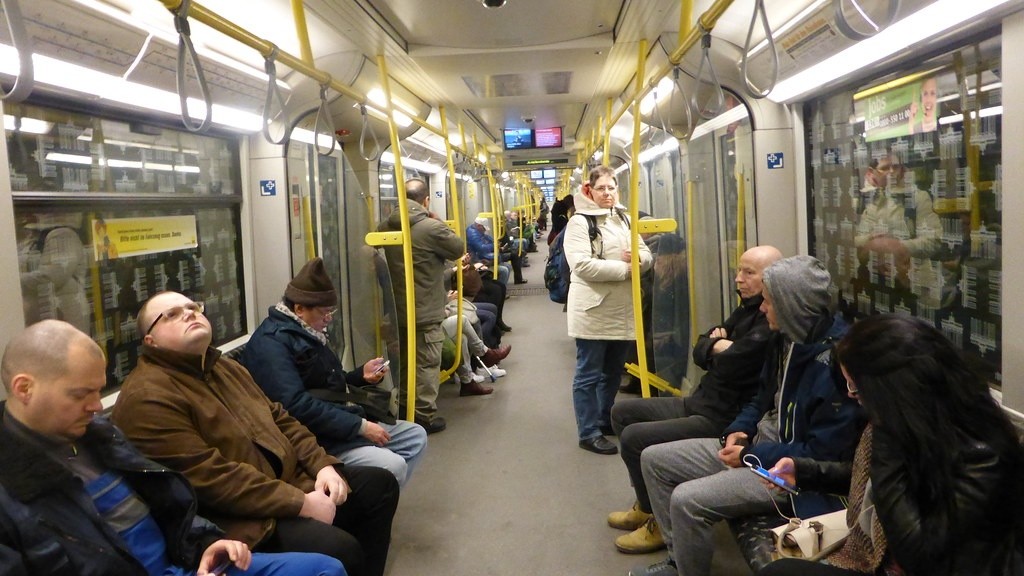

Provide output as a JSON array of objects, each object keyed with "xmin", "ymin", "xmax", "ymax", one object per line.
[{"xmin": 543, "ymin": 213, "xmax": 630, "ymax": 304}]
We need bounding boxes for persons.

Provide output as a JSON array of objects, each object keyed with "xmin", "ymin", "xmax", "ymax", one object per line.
[
  {"xmin": 909, "ymin": 78, "xmax": 936, "ymax": 134},
  {"xmin": 110, "ymin": 291, "xmax": 399, "ymax": 576},
  {"xmin": 467, "ymin": 218, "xmax": 527, "ymax": 299},
  {"xmin": 551, "ymin": 194, "xmax": 575, "ymax": 234},
  {"xmin": 755, "ymin": 316, "xmax": 1024, "ymax": 576},
  {"xmin": 607, "ymin": 246, "xmax": 783, "ymax": 552},
  {"xmin": 442, "ymin": 253, "xmax": 513, "ymax": 395},
  {"xmin": 640, "ymin": 255, "xmax": 866, "ymax": 576},
  {"xmin": 241, "ymin": 256, "xmax": 427, "ymax": 488},
  {"xmin": 850, "ymin": 152, "xmax": 945, "ymax": 313},
  {"xmin": 375, "ymin": 179, "xmax": 464, "ymax": 432},
  {"xmin": 564, "ymin": 168, "xmax": 653, "ymax": 455},
  {"xmin": 619, "ymin": 211, "xmax": 688, "ymax": 396},
  {"xmin": 19, "ymin": 186, "xmax": 91, "ymax": 338},
  {"xmin": 501, "ymin": 196, "xmax": 548, "ymax": 267},
  {"xmin": 0, "ymin": 320, "xmax": 347, "ymax": 576}
]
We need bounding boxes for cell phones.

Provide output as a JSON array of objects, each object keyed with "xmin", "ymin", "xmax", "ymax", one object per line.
[
  {"xmin": 461, "ymin": 252, "xmax": 474, "ymax": 262},
  {"xmin": 211, "ymin": 560, "xmax": 234, "ymax": 576},
  {"xmin": 370, "ymin": 360, "xmax": 389, "ymax": 376},
  {"xmin": 751, "ymin": 463, "xmax": 801, "ymax": 496}
]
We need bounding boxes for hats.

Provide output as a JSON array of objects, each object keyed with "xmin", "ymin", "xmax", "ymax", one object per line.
[
  {"xmin": 285, "ymin": 257, "xmax": 338, "ymax": 306},
  {"xmin": 475, "ymin": 217, "xmax": 490, "ymax": 232}
]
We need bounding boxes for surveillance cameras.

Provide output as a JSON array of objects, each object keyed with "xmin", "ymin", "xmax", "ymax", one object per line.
[{"xmin": 478, "ymin": 0, "xmax": 506, "ymax": 9}]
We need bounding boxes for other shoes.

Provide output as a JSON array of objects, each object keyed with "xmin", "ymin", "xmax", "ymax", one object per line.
[
  {"xmin": 620, "ymin": 382, "xmax": 641, "ymax": 394},
  {"xmin": 499, "ymin": 321, "xmax": 512, "ymax": 331},
  {"xmin": 516, "ymin": 279, "xmax": 527, "ymax": 284},
  {"xmin": 425, "ymin": 417, "xmax": 446, "ymax": 433}
]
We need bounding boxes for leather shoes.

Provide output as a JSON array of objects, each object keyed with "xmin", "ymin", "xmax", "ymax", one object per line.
[
  {"xmin": 601, "ymin": 426, "xmax": 615, "ymax": 434},
  {"xmin": 579, "ymin": 435, "xmax": 618, "ymax": 454},
  {"xmin": 481, "ymin": 344, "xmax": 510, "ymax": 367},
  {"xmin": 460, "ymin": 379, "xmax": 493, "ymax": 396}
]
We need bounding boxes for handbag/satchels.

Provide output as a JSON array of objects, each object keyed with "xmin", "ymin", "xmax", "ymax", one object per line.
[
  {"xmin": 354, "ymin": 382, "xmax": 392, "ymax": 424},
  {"xmin": 771, "ymin": 508, "xmax": 850, "ymax": 562},
  {"xmin": 447, "ymin": 298, "xmax": 480, "ymax": 324}
]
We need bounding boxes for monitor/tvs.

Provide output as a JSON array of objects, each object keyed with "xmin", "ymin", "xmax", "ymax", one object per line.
[{"xmin": 504, "ymin": 126, "xmax": 562, "ymax": 150}]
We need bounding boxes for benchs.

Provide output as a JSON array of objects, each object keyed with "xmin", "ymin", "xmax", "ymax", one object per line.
[{"xmin": 727, "ymin": 512, "xmax": 801, "ymax": 567}]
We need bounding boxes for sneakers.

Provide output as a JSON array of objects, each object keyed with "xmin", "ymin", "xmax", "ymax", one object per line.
[
  {"xmin": 628, "ymin": 557, "xmax": 678, "ymax": 576},
  {"xmin": 454, "ymin": 371, "xmax": 484, "ymax": 384},
  {"xmin": 608, "ymin": 502, "xmax": 651, "ymax": 530},
  {"xmin": 615, "ymin": 515, "xmax": 666, "ymax": 553},
  {"xmin": 476, "ymin": 364, "xmax": 506, "ymax": 377}
]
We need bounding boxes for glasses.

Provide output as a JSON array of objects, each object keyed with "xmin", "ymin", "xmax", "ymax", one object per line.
[
  {"xmin": 147, "ymin": 302, "xmax": 204, "ymax": 334},
  {"xmin": 316, "ymin": 307, "xmax": 338, "ymax": 319},
  {"xmin": 593, "ymin": 186, "xmax": 616, "ymax": 192},
  {"xmin": 846, "ymin": 381, "xmax": 860, "ymax": 396}
]
